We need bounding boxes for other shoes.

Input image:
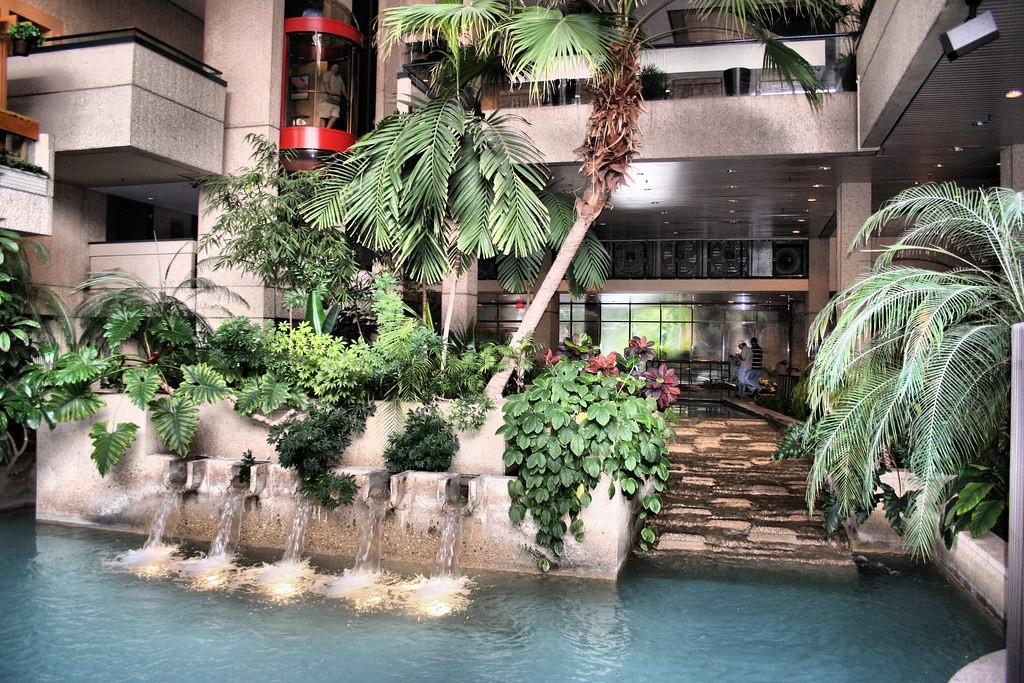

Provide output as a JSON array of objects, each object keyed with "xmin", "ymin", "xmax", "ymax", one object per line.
[
  {"xmin": 734, "ymin": 394, "xmax": 741, "ymax": 399},
  {"xmin": 759, "ymin": 389, "xmax": 765, "ymax": 394},
  {"xmin": 752, "ymin": 389, "xmax": 758, "ymax": 397}
]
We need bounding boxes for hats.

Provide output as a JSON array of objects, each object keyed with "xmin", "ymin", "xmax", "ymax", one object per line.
[
  {"xmin": 737, "ymin": 340, "xmax": 744, "ymax": 346},
  {"xmin": 751, "ymin": 337, "xmax": 757, "ymax": 343}
]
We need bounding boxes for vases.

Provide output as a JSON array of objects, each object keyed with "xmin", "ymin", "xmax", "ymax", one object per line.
[{"xmin": 723, "ymin": 66, "xmax": 753, "ymax": 97}]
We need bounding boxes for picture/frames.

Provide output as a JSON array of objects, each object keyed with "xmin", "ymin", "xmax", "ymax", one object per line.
[{"xmin": 289, "ymin": 74, "xmax": 310, "ymax": 101}]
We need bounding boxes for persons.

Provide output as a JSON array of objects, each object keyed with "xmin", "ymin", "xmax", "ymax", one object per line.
[
  {"xmin": 318, "ymin": 63, "xmax": 349, "ymax": 128},
  {"xmin": 750, "ymin": 337, "xmax": 765, "ymax": 392},
  {"xmin": 736, "ymin": 340, "xmax": 758, "ymax": 399}
]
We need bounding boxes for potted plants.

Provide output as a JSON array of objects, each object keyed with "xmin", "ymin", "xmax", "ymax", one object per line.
[
  {"xmin": 8, "ymin": 23, "xmax": 45, "ymax": 54},
  {"xmin": 836, "ymin": 52, "xmax": 857, "ymax": 92}
]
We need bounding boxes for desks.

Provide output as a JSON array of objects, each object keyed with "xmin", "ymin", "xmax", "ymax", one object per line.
[{"xmin": 292, "ymin": 114, "xmax": 310, "ymax": 126}]
